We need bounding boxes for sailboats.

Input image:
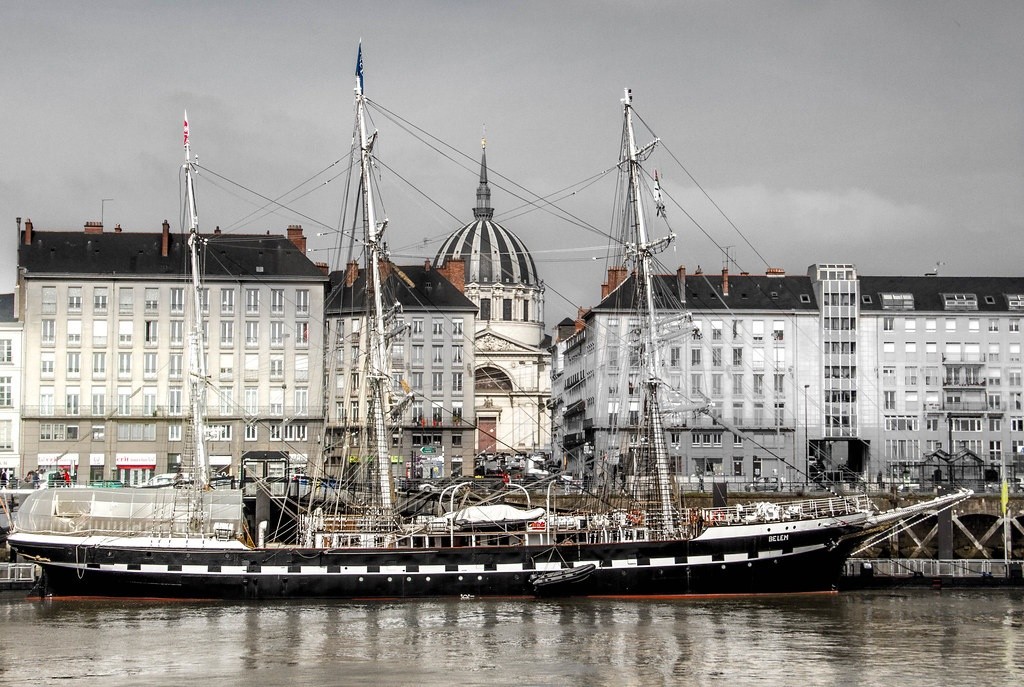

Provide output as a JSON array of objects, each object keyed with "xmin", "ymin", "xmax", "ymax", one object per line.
[{"xmin": 4, "ymin": 37, "xmax": 982, "ymax": 597}]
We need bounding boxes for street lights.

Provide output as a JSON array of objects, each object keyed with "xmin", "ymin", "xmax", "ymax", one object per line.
[
  {"xmin": 281, "ymin": 382, "xmax": 288, "ymax": 452},
  {"xmin": 802, "ymin": 384, "xmax": 809, "ymax": 487}
]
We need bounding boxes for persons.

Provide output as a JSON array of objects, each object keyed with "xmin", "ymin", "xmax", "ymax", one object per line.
[{"xmin": 0, "ymin": 467, "xmax": 71, "ymax": 488}]
